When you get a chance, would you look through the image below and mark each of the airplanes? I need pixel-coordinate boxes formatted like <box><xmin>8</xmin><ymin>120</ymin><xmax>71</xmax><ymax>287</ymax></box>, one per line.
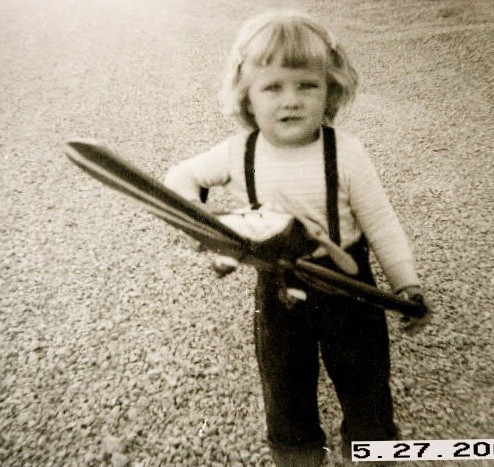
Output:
<box><xmin>61</xmin><ymin>137</ymin><xmax>429</xmax><ymax>321</ymax></box>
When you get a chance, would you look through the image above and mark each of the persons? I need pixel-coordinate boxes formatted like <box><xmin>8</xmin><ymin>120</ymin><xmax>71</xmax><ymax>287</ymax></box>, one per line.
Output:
<box><xmin>162</xmin><ymin>11</ymin><xmax>432</xmax><ymax>467</ymax></box>
